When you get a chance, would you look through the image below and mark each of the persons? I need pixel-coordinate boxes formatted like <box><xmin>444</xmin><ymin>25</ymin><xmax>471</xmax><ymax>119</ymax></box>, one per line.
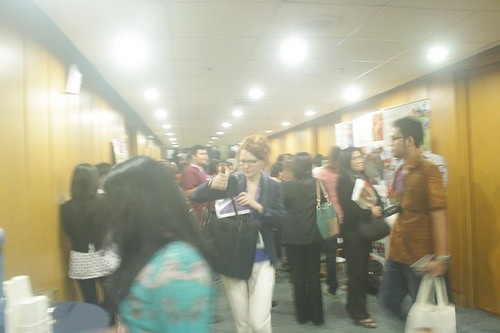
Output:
<box><xmin>60</xmin><ymin>133</ymin><xmax>384</xmax><ymax>333</ymax></box>
<box><xmin>379</xmin><ymin>116</ymin><xmax>457</xmax><ymax>333</ymax></box>
<box><xmin>103</xmin><ymin>155</ymin><xmax>219</xmax><ymax>333</ymax></box>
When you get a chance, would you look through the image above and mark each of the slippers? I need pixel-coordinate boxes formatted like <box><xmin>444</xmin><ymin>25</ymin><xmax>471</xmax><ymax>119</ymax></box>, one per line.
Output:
<box><xmin>353</xmin><ymin>315</ymin><xmax>377</xmax><ymax>329</ymax></box>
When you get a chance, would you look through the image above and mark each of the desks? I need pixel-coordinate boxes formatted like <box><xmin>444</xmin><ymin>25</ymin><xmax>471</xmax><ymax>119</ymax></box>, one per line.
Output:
<box><xmin>0</xmin><ymin>300</ymin><xmax>109</xmax><ymax>333</ymax></box>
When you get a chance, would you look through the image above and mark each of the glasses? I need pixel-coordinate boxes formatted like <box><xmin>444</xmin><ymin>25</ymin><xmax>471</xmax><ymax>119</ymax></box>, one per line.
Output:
<box><xmin>392</xmin><ymin>136</ymin><xmax>405</xmax><ymax>142</ymax></box>
<box><xmin>237</xmin><ymin>158</ymin><xmax>260</xmax><ymax>167</ymax></box>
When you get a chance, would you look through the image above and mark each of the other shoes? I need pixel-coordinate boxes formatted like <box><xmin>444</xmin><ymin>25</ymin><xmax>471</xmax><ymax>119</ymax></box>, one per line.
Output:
<box><xmin>326</xmin><ymin>286</ymin><xmax>344</xmax><ymax>296</ymax></box>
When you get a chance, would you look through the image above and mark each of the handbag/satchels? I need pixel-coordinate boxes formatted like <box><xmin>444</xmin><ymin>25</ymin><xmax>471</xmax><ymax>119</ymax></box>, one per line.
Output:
<box><xmin>315</xmin><ymin>179</ymin><xmax>340</xmax><ymax>241</ymax></box>
<box><xmin>357</xmin><ymin>217</ymin><xmax>391</xmax><ymax>241</ymax></box>
<box><xmin>404</xmin><ymin>276</ymin><xmax>457</xmax><ymax>332</ymax></box>
<box><xmin>199</xmin><ymin>191</ymin><xmax>259</xmax><ymax>280</ymax></box>
